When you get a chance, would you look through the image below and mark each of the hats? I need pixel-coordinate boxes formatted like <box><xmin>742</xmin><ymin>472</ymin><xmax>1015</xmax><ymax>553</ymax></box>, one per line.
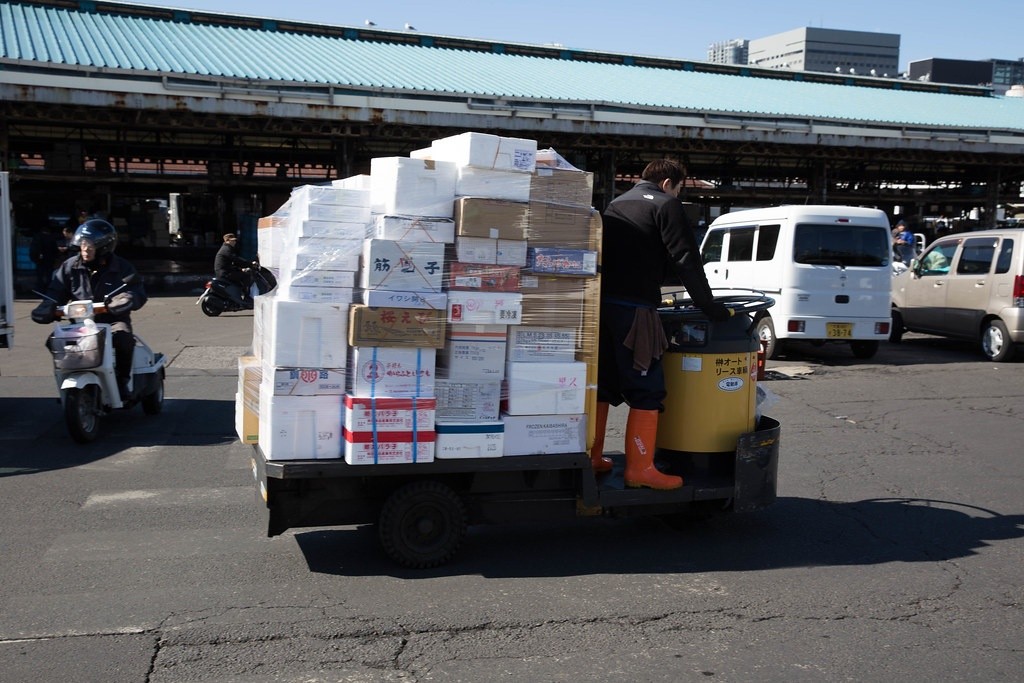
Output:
<box><xmin>223</xmin><ymin>233</ymin><xmax>237</xmax><ymax>241</ymax></box>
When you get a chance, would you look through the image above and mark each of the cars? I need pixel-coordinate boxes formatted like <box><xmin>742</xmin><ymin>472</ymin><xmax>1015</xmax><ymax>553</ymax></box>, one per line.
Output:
<box><xmin>1005</xmin><ymin>218</ymin><xmax>1024</xmax><ymax>228</ymax></box>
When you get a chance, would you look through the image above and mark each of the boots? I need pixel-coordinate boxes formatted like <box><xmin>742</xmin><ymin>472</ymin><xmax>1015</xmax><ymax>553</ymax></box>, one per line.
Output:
<box><xmin>624</xmin><ymin>408</ymin><xmax>683</xmax><ymax>490</ymax></box>
<box><xmin>112</xmin><ymin>367</ymin><xmax>133</xmax><ymax>404</ymax></box>
<box><xmin>592</xmin><ymin>402</ymin><xmax>614</xmax><ymax>471</ymax></box>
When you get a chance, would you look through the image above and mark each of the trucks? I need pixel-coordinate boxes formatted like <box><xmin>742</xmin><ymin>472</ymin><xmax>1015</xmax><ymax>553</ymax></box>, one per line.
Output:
<box><xmin>250</xmin><ymin>286</ymin><xmax>782</xmax><ymax>569</ymax></box>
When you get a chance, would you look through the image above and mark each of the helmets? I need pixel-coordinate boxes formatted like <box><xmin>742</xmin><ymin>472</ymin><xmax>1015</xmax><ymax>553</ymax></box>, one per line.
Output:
<box><xmin>77</xmin><ymin>219</ymin><xmax>117</xmax><ymax>259</ymax></box>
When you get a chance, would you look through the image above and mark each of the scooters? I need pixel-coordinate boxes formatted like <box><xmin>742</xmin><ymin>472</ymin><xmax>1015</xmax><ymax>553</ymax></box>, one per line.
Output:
<box><xmin>196</xmin><ymin>261</ymin><xmax>273</xmax><ymax>317</ymax></box>
<box><xmin>18</xmin><ymin>274</ymin><xmax>167</xmax><ymax>442</ymax></box>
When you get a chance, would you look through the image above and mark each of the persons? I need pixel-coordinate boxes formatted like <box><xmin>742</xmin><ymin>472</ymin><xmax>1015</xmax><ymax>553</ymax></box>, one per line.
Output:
<box><xmin>31</xmin><ymin>230</ymin><xmax>52</xmax><ymax>298</ymax></box>
<box><xmin>33</xmin><ymin>219</ymin><xmax>146</xmax><ymax>402</ymax></box>
<box><xmin>214</xmin><ymin>234</ymin><xmax>258</xmax><ymax>305</ymax></box>
<box><xmin>589</xmin><ymin>158</ymin><xmax>730</xmax><ymax>491</ymax></box>
<box><xmin>57</xmin><ymin>227</ymin><xmax>80</xmax><ymax>256</ymax></box>
<box><xmin>79</xmin><ymin>212</ymin><xmax>87</xmax><ymax>223</ymax></box>
<box><xmin>891</xmin><ymin>220</ymin><xmax>915</xmax><ymax>267</ymax></box>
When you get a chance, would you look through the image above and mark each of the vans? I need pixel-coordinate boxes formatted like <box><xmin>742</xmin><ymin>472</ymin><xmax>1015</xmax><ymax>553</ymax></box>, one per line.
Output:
<box><xmin>892</xmin><ymin>228</ymin><xmax>1024</xmax><ymax>362</ymax></box>
<box><xmin>681</xmin><ymin>205</ymin><xmax>893</xmax><ymax>359</ymax></box>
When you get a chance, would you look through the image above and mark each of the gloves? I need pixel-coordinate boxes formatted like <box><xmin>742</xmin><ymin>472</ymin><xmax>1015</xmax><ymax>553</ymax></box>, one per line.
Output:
<box><xmin>704</xmin><ymin>303</ymin><xmax>731</xmax><ymax>323</ymax></box>
